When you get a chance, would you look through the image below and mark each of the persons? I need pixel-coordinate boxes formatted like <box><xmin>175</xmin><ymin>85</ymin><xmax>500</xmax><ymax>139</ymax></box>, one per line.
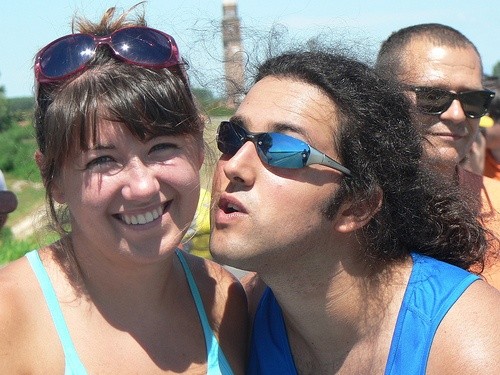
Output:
<box><xmin>374</xmin><ymin>24</ymin><xmax>500</xmax><ymax>291</ymax></box>
<box><xmin>184</xmin><ymin>18</ymin><xmax>500</xmax><ymax>375</ymax></box>
<box><xmin>0</xmin><ymin>0</ymin><xmax>249</xmax><ymax>375</ymax></box>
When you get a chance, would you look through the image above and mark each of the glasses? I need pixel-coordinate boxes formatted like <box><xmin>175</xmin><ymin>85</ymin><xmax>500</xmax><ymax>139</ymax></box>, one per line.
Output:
<box><xmin>216</xmin><ymin>121</ymin><xmax>354</xmax><ymax>177</ymax></box>
<box><xmin>485</xmin><ymin>100</ymin><xmax>500</xmax><ymax>121</ymax></box>
<box><xmin>396</xmin><ymin>83</ymin><xmax>495</xmax><ymax>119</ymax></box>
<box><xmin>34</xmin><ymin>27</ymin><xmax>193</xmax><ymax>103</ymax></box>
